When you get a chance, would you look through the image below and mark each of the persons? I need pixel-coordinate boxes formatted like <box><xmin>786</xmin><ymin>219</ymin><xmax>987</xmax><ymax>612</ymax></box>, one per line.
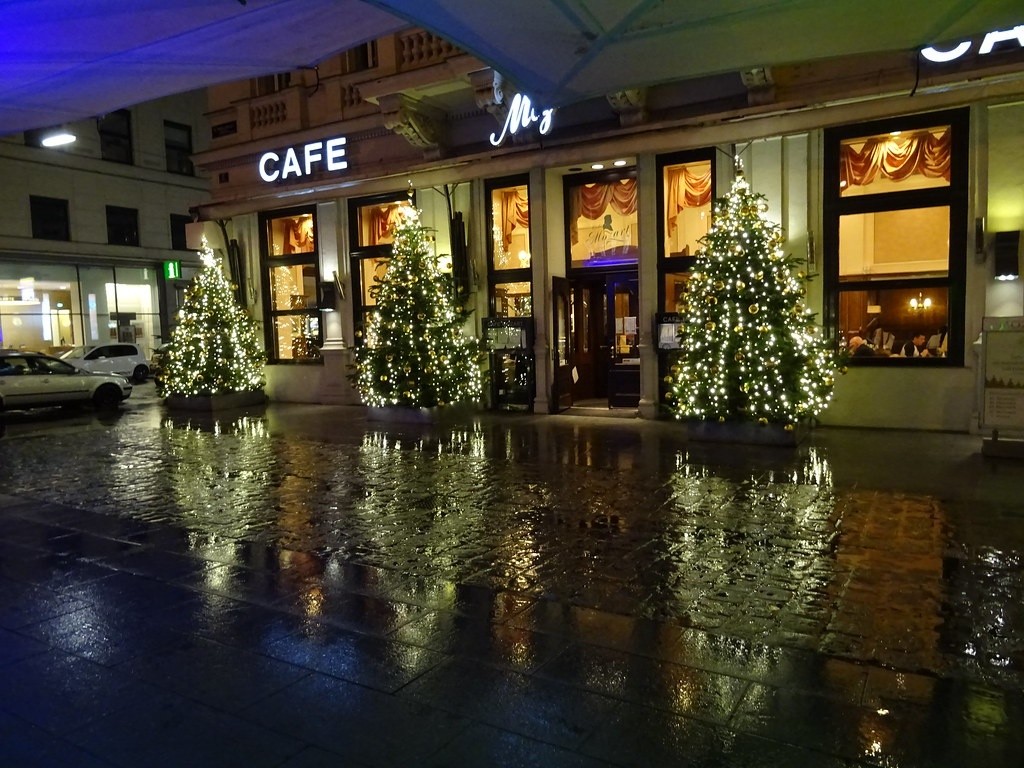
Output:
<box><xmin>849</xmin><ymin>336</ymin><xmax>875</xmax><ymax>356</ymax></box>
<box><xmin>900</xmin><ymin>332</ymin><xmax>928</xmax><ymax>358</ymax></box>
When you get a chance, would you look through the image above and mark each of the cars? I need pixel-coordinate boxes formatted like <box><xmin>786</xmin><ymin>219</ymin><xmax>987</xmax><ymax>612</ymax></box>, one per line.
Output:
<box><xmin>22</xmin><ymin>342</ymin><xmax>150</xmax><ymax>385</ymax></box>
<box><xmin>149</xmin><ymin>343</ymin><xmax>170</xmax><ymax>370</ymax></box>
<box><xmin>0</xmin><ymin>350</ymin><xmax>133</xmax><ymax>414</ymax></box>
